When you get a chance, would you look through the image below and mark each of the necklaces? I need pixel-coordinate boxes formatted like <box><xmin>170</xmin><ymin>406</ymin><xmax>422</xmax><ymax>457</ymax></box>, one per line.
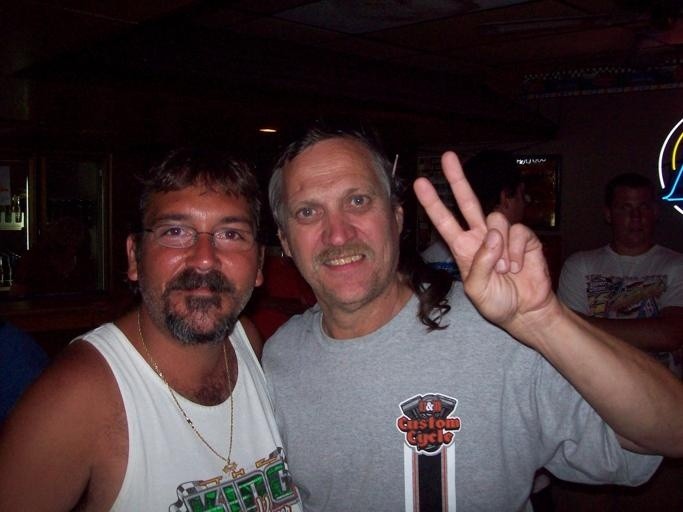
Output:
<box><xmin>137</xmin><ymin>306</ymin><xmax>237</xmax><ymax>474</ymax></box>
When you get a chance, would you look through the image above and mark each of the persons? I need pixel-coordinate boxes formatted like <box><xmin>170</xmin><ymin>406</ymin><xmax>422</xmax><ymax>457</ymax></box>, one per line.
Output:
<box><xmin>420</xmin><ymin>149</ymin><xmax>530</xmax><ymax>287</ymax></box>
<box><xmin>1</xmin><ymin>142</ymin><xmax>302</xmax><ymax>512</ymax></box>
<box><xmin>258</xmin><ymin>120</ymin><xmax>683</xmax><ymax>512</ymax></box>
<box><xmin>556</xmin><ymin>171</ymin><xmax>683</xmax><ymax>380</ymax></box>
<box><xmin>0</xmin><ymin>313</ymin><xmax>49</xmax><ymax>418</ymax></box>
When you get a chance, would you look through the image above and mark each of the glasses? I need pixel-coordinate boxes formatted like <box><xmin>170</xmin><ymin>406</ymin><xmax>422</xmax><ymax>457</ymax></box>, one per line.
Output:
<box><xmin>144</xmin><ymin>223</ymin><xmax>255</xmax><ymax>250</ymax></box>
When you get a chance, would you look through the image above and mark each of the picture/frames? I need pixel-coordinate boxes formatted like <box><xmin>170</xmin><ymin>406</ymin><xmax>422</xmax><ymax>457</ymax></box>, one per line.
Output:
<box><xmin>509</xmin><ymin>153</ymin><xmax>562</xmax><ymax>233</ymax></box>
